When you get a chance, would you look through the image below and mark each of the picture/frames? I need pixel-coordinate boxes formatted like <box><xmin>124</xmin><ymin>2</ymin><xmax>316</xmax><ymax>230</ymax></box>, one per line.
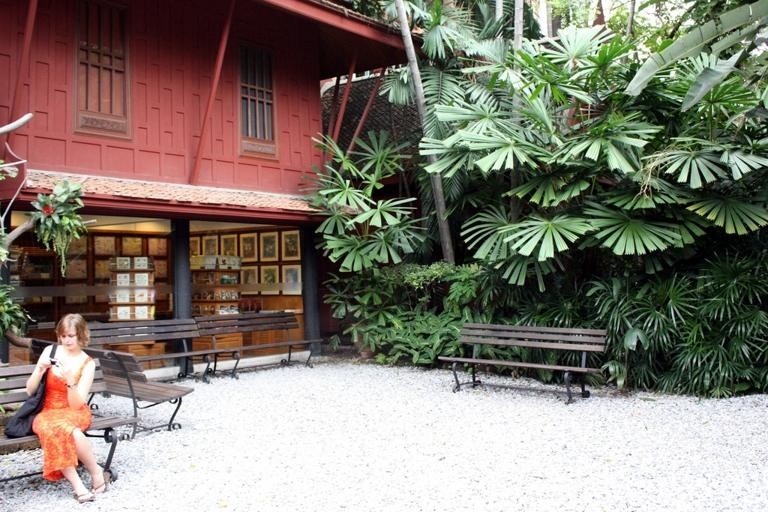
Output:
<box><xmin>57</xmin><ymin>230</ymin><xmax>304</xmax><ymax>307</ymax></box>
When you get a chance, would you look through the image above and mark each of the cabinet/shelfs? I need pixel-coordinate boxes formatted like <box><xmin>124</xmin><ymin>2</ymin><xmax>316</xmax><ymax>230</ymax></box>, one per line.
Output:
<box><xmin>184</xmin><ymin>268</ymin><xmax>249</xmax><ymax>358</ymax></box>
<box><xmin>19</xmin><ymin>246</ymin><xmax>57</xmax><ymax>341</ymax></box>
<box><xmin>102</xmin><ymin>264</ymin><xmax>170</xmax><ymax>368</ymax></box>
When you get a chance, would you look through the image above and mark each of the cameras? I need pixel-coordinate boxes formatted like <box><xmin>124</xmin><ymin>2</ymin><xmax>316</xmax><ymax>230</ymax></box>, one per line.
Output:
<box><xmin>49</xmin><ymin>359</ymin><xmax>57</xmax><ymax>365</ymax></box>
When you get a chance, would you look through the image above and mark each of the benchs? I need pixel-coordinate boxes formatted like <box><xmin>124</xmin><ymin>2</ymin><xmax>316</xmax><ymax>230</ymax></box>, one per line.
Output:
<box><xmin>19</xmin><ymin>333</ymin><xmax>195</xmax><ymax>441</ymax></box>
<box><xmin>84</xmin><ymin>314</ymin><xmax>218</xmax><ymax>383</ymax></box>
<box><xmin>435</xmin><ymin>318</ymin><xmax>610</xmax><ymax>406</ymax></box>
<box><xmin>0</xmin><ymin>356</ymin><xmax>143</xmax><ymax>483</ymax></box>
<box><xmin>192</xmin><ymin>307</ymin><xmax>326</xmax><ymax>379</ymax></box>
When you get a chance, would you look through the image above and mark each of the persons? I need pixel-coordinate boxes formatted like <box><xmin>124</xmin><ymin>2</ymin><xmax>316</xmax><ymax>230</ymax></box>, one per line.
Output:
<box><xmin>26</xmin><ymin>313</ymin><xmax>111</xmax><ymax>502</ymax></box>
<box><xmin>188</xmin><ymin>230</ymin><xmax>299</xmax><ymax>294</ymax></box>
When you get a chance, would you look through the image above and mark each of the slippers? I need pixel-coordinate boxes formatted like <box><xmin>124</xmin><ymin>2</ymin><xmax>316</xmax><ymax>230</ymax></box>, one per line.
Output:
<box><xmin>73</xmin><ymin>489</ymin><xmax>96</xmax><ymax>504</ymax></box>
<box><xmin>91</xmin><ymin>469</ymin><xmax>111</xmax><ymax>495</ymax></box>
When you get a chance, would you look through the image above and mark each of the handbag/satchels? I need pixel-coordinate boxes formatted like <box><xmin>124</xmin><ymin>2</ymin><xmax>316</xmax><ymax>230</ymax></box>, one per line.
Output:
<box><xmin>2</xmin><ymin>342</ymin><xmax>59</xmax><ymax>439</ymax></box>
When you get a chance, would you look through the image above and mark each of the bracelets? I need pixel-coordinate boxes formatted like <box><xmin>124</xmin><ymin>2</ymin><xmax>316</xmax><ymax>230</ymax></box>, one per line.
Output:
<box><xmin>64</xmin><ymin>382</ymin><xmax>79</xmax><ymax>389</ymax></box>
